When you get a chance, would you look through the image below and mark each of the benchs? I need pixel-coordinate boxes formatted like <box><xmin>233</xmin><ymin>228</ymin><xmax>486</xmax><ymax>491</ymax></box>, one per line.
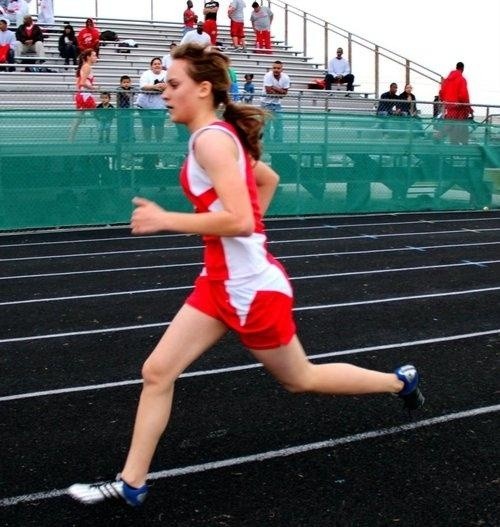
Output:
<box><xmin>1</xmin><ymin>14</ymin><xmax>426</xmax><ymax>146</ymax></box>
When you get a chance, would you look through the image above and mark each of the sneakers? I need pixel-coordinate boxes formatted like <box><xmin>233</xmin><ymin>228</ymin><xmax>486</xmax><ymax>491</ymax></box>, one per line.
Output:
<box><xmin>68</xmin><ymin>472</ymin><xmax>149</xmax><ymax>508</ymax></box>
<box><xmin>392</xmin><ymin>364</ymin><xmax>426</xmax><ymax>410</ymax></box>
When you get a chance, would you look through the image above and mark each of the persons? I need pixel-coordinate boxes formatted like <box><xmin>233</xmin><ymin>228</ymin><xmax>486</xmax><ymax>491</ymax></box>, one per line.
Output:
<box><xmin>374</xmin><ymin>61</ymin><xmax>475</xmax><ymax>148</ymax></box>
<box><xmin>76</xmin><ymin>18</ymin><xmax>102</xmax><ymax>62</ymax></box>
<box><xmin>67</xmin><ymin>48</ymin><xmax>99</xmax><ymax>143</ymax></box>
<box><xmin>115</xmin><ymin>0</ymin><xmax>291</xmax><ymax>143</ymax></box>
<box><xmin>323</xmin><ymin>47</ymin><xmax>354</xmax><ymax>96</ymax></box>
<box><xmin>64</xmin><ymin>41</ymin><xmax>434</xmax><ymax>506</ymax></box>
<box><xmin>93</xmin><ymin>92</ymin><xmax>116</xmax><ymax>146</ymax></box>
<box><xmin>0</xmin><ymin>0</ymin><xmax>55</xmax><ymax>72</ymax></box>
<box><xmin>58</xmin><ymin>25</ymin><xmax>79</xmax><ymax>71</ymax></box>
